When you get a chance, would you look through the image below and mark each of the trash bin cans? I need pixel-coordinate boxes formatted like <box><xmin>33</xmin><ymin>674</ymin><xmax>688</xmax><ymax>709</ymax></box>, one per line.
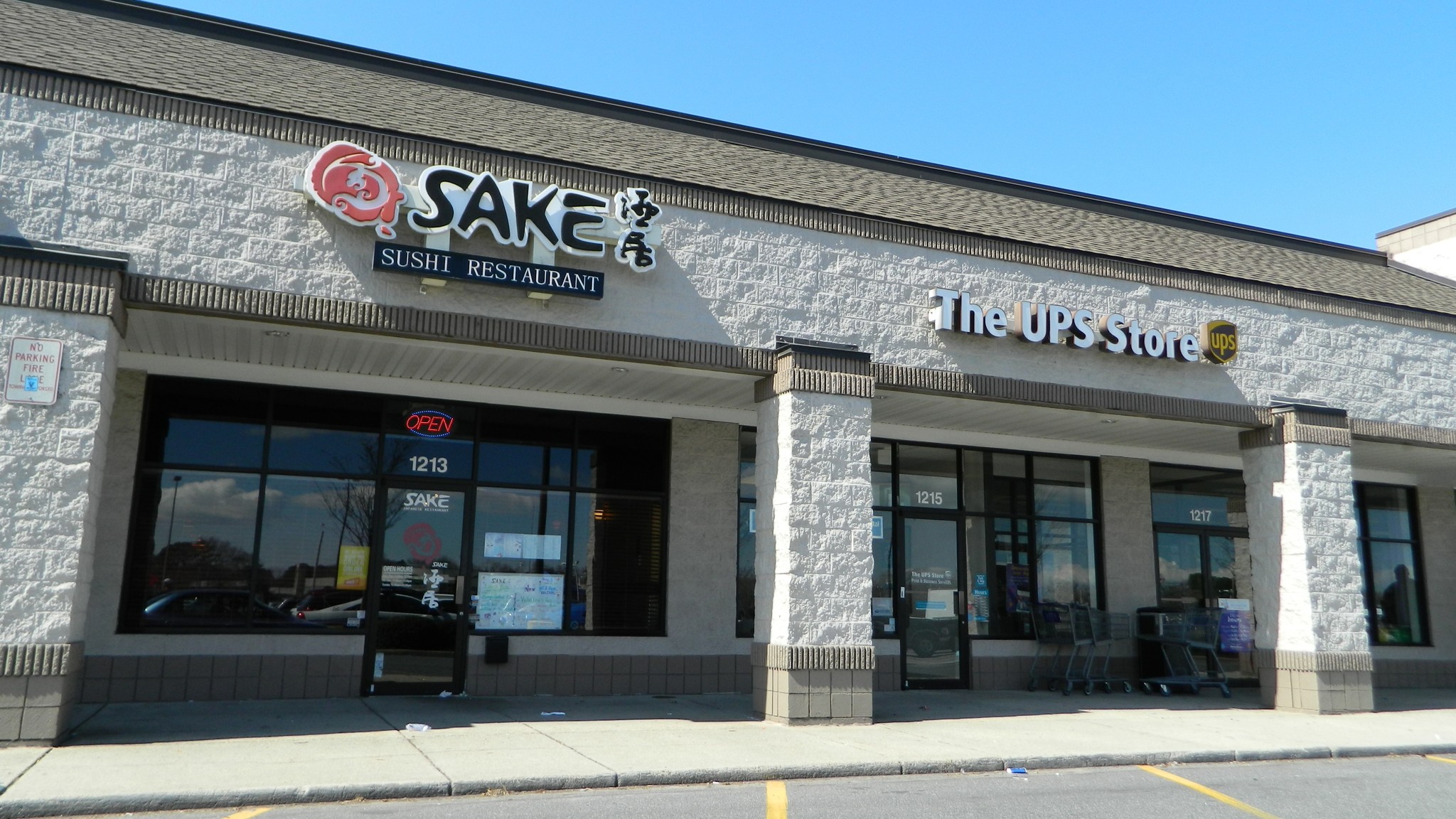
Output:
<box><xmin>1137</xmin><ymin>607</ymin><xmax>1188</xmax><ymax>693</ymax></box>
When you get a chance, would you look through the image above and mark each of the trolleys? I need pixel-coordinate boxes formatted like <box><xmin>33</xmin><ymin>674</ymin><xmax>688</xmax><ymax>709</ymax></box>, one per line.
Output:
<box><xmin>1135</xmin><ymin>608</ymin><xmax>1232</xmax><ymax>698</ymax></box>
<box><xmin>1063</xmin><ymin>602</ymin><xmax>1134</xmax><ymax>696</ymax></box>
<box><xmin>1026</xmin><ymin>601</ymin><xmax>1080</xmax><ymax>691</ymax></box>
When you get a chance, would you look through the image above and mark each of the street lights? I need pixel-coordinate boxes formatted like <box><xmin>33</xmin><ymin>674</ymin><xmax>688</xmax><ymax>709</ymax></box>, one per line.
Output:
<box><xmin>312</xmin><ymin>523</ymin><xmax>325</xmax><ymax>588</ymax></box>
<box><xmin>159</xmin><ymin>476</ymin><xmax>182</xmax><ymax>592</ymax></box>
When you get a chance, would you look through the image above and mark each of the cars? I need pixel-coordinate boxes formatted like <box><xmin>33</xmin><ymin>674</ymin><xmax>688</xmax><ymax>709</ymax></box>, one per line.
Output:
<box><xmin>471</xmin><ymin>594</ymin><xmax>586</xmax><ymax>631</ymax></box>
<box><xmin>143</xmin><ymin>590</ymin><xmax>292</xmax><ymax>628</ymax></box>
<box><xmin>269</xmin><ymin>588</ymin><xmax>509</xmax><ymax>655</ymax></box>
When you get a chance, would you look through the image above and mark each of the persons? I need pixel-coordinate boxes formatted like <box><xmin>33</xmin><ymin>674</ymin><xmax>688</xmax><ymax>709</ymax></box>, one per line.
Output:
<box><xmin>1381</xmin><ymin>564</ymin><xmax>1418</xmax><ymax>643</ymax></box>
